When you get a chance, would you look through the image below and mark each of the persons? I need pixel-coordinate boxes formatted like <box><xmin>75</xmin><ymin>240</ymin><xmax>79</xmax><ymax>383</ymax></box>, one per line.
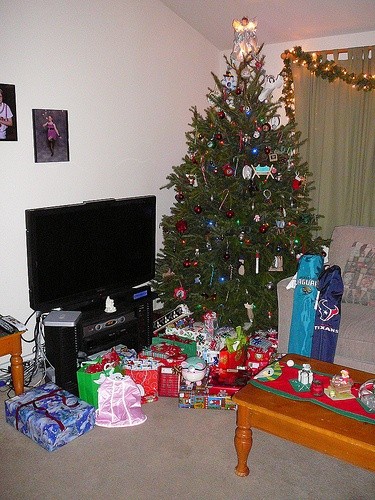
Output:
<box><xmin>0</xmin><ymin>89</ymin><xmax>13</xmax><ymax>140</ymax></box>
<box><xmin>43</xmin><ymin>115</ymin><xmax>59</xmax><ymax>156</ymax></box>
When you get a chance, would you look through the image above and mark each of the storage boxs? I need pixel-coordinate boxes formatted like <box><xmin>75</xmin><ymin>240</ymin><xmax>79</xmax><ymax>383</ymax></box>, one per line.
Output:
<box><xmin>5</xmin><ymin>313</ymin><xmax>275</xmax><ymax>453</ymax></box>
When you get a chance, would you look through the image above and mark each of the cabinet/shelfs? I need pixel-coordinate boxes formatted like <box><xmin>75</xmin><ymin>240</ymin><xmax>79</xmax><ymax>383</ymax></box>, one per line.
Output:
<box><xmin>77</xmin><ymin>293</ymin><xmax>160</xmax><ymax>354</ymax></box>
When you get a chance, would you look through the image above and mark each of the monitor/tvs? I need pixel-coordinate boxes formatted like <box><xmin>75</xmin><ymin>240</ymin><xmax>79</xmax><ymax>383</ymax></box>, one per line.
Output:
<box><xmin>26</xmin><ymin>195</ymin><xmax>156</xmax><ymax>311</ymax></box>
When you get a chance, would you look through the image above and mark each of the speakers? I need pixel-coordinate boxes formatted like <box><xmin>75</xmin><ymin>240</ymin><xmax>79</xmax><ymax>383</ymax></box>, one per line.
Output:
<box><xmin>43</xmin><ymin>320</ymin><xmax>83</xmax><ymax>387</ymax></box>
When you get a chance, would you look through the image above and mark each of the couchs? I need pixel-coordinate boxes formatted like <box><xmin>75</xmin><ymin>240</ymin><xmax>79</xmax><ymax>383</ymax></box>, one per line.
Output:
<box><xmin>277</xmin><ymin>226</ymin><xmax>375</xmax><ymax>373</ymax></box>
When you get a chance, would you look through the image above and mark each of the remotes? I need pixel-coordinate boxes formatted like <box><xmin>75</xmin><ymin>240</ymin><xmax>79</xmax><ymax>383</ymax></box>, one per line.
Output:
<box><xmin>0</xmin><ymin>319</ymin><xmax>14</xmax><ymax>333</ymax></box>
<box><xmin>1</xmin><ymin>316</ymin><xmax>26</xmax><ymax>331</ymax></box>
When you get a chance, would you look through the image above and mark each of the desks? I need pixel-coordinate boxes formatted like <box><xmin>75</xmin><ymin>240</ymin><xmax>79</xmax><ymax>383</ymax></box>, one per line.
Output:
<box><xmin>231</xmin><ymin>353</ymin><xmax>375</xmax><ymax>477</ymax></box>
<box><xmin>0</xmin><ymin>313</ymin><xmax>26</xmax><ymax>398</ymax></box>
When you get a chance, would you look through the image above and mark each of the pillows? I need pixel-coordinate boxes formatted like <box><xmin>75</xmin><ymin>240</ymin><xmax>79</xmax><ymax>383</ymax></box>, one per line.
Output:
<box><xmin>340</xmin><ymin>241</ymin><xmax>375</xmax><ymax>307</ymax></box>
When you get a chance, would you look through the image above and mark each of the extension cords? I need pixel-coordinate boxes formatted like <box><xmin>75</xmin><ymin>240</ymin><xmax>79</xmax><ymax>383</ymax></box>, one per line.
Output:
<box><xmin>44</xmin><ymin>367</ymin><xmax>56</xmax><ymax>383</ymax></box>
